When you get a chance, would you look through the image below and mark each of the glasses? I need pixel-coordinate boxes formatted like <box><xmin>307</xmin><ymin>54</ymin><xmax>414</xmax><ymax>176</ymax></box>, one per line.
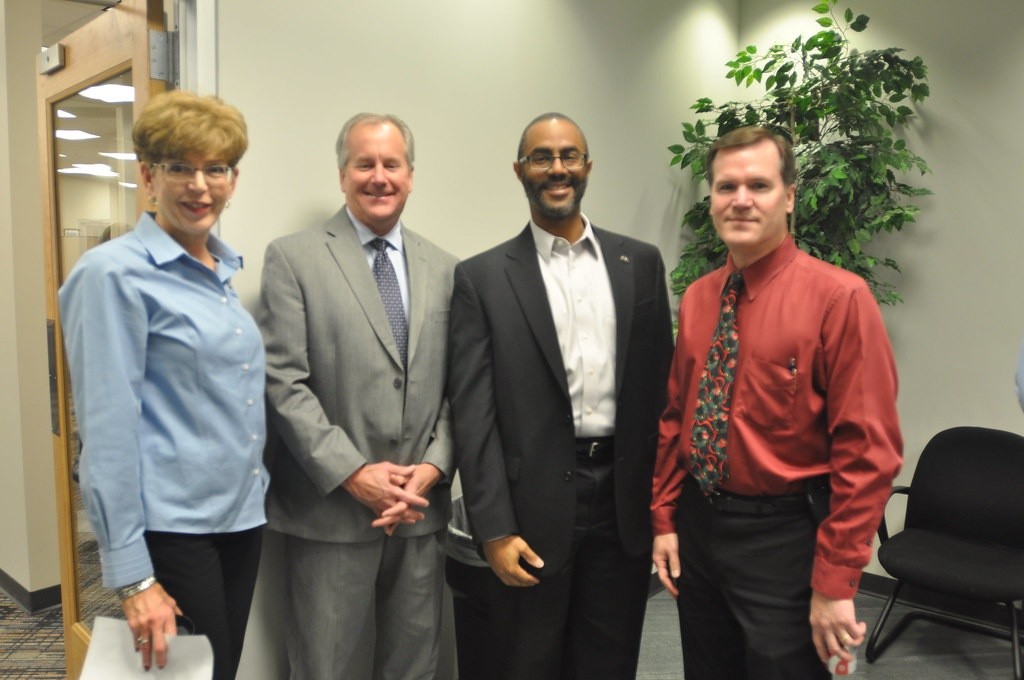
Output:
<box><xmin>151</xmin><ymin>159</ymin><xmax>237</xmax><ymax>186</ymax></box>
<box><xmin>517</xmin><ymin>153</ymin><xmax>591</xmax><ymax>173</ymax></box>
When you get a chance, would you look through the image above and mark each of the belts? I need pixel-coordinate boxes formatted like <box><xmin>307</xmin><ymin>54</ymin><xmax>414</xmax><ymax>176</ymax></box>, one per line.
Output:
<box><xmin>687</xmin><ymin>477</ymin><xmax>831</xmax><ymax>515</ymax></box>
<box><xmin>577</xmin><ymin>439</ymin><xmax>615</xmax><ymax>458</ymax></box>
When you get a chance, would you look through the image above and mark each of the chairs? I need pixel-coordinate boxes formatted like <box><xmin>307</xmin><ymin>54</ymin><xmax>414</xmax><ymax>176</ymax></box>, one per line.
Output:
<box><xmin>865</xmin><ymin>426</ymin><xmax>1024</xmax><ymax>680</ymax></box>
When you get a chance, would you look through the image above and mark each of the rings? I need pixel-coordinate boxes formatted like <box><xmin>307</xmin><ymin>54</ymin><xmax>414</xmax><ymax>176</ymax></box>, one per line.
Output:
<box><xmin>840</xmin><ymin>635</ymin><xmax>850</xmax><ymax>642</ymax></box>
<box><xmin>137</xmin><ymin>637</ymin><xmax>150</xmax><ymax>645</ymax></box>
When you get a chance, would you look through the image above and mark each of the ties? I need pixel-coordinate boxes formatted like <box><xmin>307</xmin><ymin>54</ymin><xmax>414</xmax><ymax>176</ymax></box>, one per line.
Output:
<box><xmin>689</xmin><ymin>275</ymin><xmax>746</xmax><ymax>508</ymax></box>
<box><xmin>368</xmin><ymin>238</ymin><xmax>409</xmax><ymax>411</ymax></box>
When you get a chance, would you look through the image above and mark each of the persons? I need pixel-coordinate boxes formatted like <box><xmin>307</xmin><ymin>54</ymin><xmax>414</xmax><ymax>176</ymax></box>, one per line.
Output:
<box><xmin>259</xmin><ymin>114</ymin><xmax>459</xmax><ymax>680</ymax></box>
<box><xmin>653</xmin><ymin>128</ymin><xmax>905</xmax><ymax>679</ymax></box>
<box><xmin>59</xmin><ymin>95</ymin><xmax>270</xmax><ymax>680</ymax></box>
<box><xmin>449</xmin><ymin>114</ymin><xmax>674</xmax><ymax>679</ymax></box>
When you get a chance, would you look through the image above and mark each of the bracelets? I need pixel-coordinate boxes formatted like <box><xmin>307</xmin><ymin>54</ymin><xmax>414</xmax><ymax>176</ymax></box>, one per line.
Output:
<box><xmin>115</xmin><ymin>576</ymin><xmax>156</xmax><ymax>600</ymax></box>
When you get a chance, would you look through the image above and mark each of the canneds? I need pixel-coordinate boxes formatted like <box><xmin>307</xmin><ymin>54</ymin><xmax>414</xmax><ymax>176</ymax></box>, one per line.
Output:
<box><xmin>827</xmin><ymin>637</ymin><xmax>858</xmax><ymax>676</ymax></box>
